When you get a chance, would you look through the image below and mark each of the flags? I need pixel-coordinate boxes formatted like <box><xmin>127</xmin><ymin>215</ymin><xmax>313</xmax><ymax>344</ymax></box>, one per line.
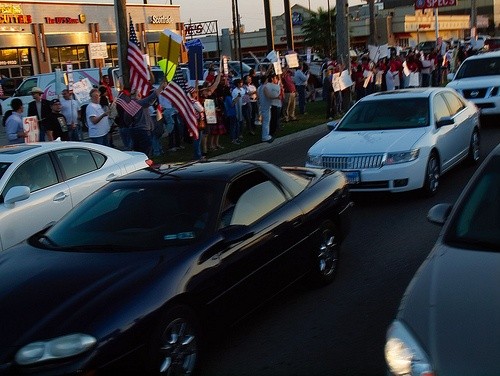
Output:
<box><xmin>160</xmin><ymin>81</ymin><xmax>200</xmax><ymax>141</ymax></box>
<box><xmin>174</xmin><ymin>62</ymin><xmax>196</xmax><ymax>94</ymax></box>
<box><xmin>127</xmin><ymin>13</ymin><xmax>150</xmax><ymax>98</ymax></box>
<box><xmin>114</xmin><ymin>92</ymin><xmax>142</xmax><ymax>117</ymax></box>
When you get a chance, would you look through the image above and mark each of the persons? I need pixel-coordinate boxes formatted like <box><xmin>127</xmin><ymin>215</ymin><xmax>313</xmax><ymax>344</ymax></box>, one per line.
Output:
<box><xmin>47</xmin><ymin>99</ymin><xmax>75</xmax><ymax>142</ymax></box>
<box><xmin>5</xmin><ymin>99</ymin><xmax>29</xmax><ymax>145</ymax></box>
<box><xmin>322</xmin><ymin>40</ymin><xmax>490</xmax><ymax>120</ymax></box>
<box><xmin>96</xmin><ymin>69</ymin><xmax>168</xmax><ymax>169</ymax></box>
<box><xmin>86</xmin><ymin>89</ymin><xmax>113</xmax><ymax>147</ymax></box>
<box><xmin>185</xmin><ymin>64</ymin><xmax>310</xmax><ymax>162</ymax></box>
<box><xmin>28</xmin><ymin>87</ymin><xmax>53</xmax><ymax>142</ymax></box>
<box><xmin>58</xmin><ymin>89</ymin><xmax>78</xmax><ymax>141</ymax></box>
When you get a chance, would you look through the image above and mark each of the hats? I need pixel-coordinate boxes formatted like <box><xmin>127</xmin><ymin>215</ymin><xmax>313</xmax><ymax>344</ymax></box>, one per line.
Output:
<box><xmin>50</xmin><ymin>99</ymin><xmax>61</xmax><ymax>105</ymax></box>
<box><xmin>28</xmin><ymin>87</ymin><xmax>44</xmax><ymax>94</ymax></box>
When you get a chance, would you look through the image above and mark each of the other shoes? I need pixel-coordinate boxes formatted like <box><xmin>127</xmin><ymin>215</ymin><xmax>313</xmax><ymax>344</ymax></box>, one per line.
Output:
<box><xmin>216</xmin><ymin>145</ymin><xmax>224</xmax><ymax>149</ymax></box>
<box><xmin>265</xmin><ymin>138</ymin><xmax>272</xmax><ymax>143</ymax></box>
<box><xmin>202</xmin><ymin>151</ymin><xmax>212</xmax><ymax>156</ymax></box>
<box><xmin>290</xmin><ymin>116</ymin><xmax>298</xmax><ymax>120</ymax></box>
<box><xmin>285</xmin><ymin>117</ymin><xmax>289</xmax><ymax>123</ymax></box>
<box><xmin>210</xmin><ymin>147</ymin><xmax>219</xmax><ymax>151</ymax></box>
<box><xmin>233</xmin><ymin>139</ymin><xmax>241</xmax><ymax>145</ymax></box>
